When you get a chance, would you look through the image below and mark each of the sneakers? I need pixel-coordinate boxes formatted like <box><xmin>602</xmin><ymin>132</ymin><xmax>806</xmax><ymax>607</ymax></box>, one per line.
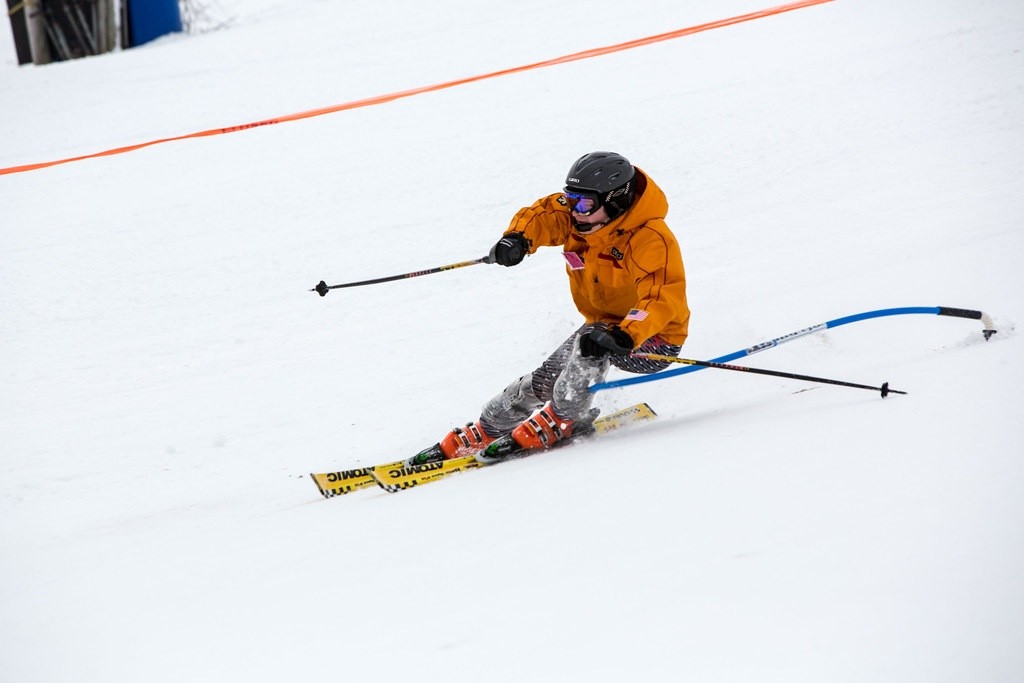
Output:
<box><xmin>441</xmin><ymin>422</ymin><xmax>498</xmax><ymax>460</ymax></box>
<box><xmin>512</xmin><ymin>406</ymin><xmax>573</xmax><ymax>451</ymax></box>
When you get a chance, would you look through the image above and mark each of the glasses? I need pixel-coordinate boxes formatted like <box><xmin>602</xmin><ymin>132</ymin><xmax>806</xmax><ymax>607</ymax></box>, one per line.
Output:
<box><xmin>563</xmin><ymin>187</ymin><xmax>601</xmax><ymax>216</ymax></box>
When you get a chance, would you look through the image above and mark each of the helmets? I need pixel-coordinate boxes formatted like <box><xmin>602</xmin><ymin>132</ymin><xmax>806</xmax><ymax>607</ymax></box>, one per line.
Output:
<box><xmin>566</xmin><ymin>151</ymin><xmax>635</xmax><ymax>219</ymax></box>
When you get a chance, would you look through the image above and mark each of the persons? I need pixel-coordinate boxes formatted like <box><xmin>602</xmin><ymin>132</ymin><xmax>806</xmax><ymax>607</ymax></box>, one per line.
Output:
<box><xmin>442</xmin><ymin>152</ymin><xmax>691</xmax><ymax>461</ymax></box>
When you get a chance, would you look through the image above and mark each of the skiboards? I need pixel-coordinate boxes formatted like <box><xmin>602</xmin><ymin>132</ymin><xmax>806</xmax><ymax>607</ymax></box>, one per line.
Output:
<box><xmin>310</xmin><ymin>403</ymin><xmax>658</xmax><ymax>499</ymax></box>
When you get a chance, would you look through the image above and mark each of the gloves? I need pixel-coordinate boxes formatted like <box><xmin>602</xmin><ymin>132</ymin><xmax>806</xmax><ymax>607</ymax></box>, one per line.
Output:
<box><xmin>579</xmin><ymin>327</ymin><xmax>634</xmax><ymax>359</ymax></box>
<box><xmin>495</xmin><ymin>233</ymin><xmax>528</xmax><ymax>267</ymax></box>
